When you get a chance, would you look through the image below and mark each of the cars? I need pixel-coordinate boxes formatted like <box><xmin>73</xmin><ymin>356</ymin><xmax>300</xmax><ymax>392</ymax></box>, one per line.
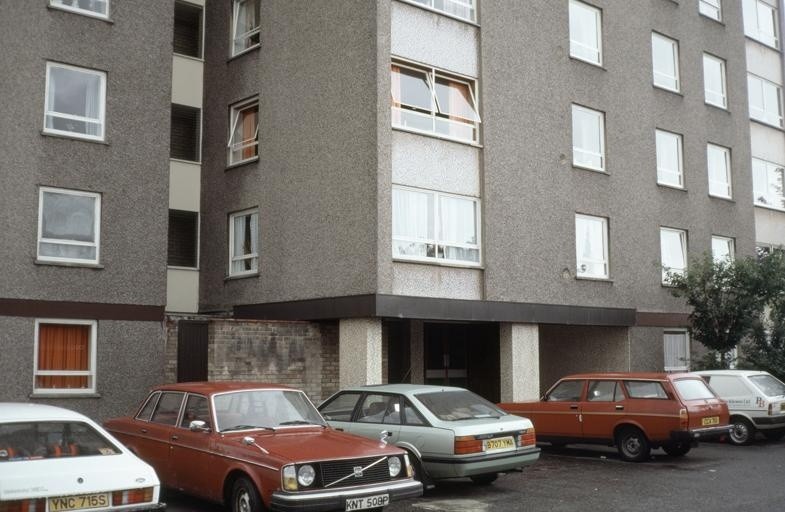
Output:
<box><xmin>0</xmin><ymin>402</ymin><xmax>167</xmax><ymax>512</ymax></box>
<box><xmin>314</xmin><ymin>382</ymin><xmax>542</xmax><ymax>498</ymax></box>
<box><xmin>495</xmin><ymin>371</ymin><xmax>735</xmax><ymax>463</ymax></box>
<box><xmin>702</xmin><ymin>366</ymin><xmax>784</xmax><ymax>443</ymax></box>
<box><xmin>100</xmin><ymin>381</ymin><xmax>425</xmax><ymax>512</ymax></box>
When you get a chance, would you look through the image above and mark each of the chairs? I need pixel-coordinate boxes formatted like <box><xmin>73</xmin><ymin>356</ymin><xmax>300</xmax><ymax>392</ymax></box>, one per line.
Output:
<box><xmin>366</xmin><ymin>402</ymin><xmax>384</xmax><ymax>415</ymax></box>
